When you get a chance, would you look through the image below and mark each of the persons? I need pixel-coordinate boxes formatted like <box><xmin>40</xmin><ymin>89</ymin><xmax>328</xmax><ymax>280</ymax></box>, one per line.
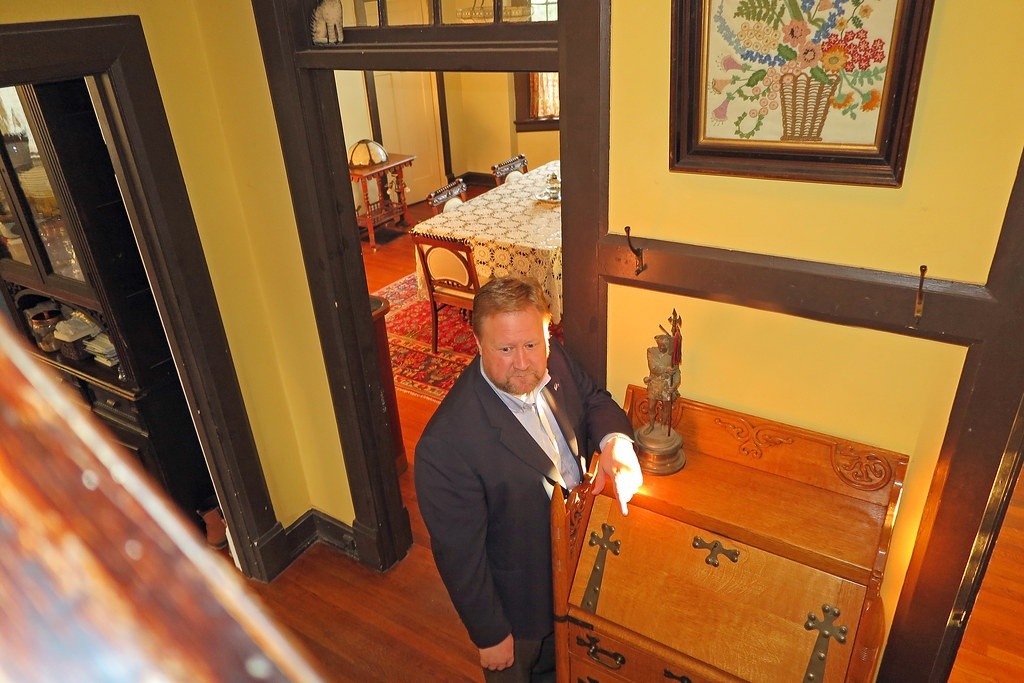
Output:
<box><xmin>416</xmin><ymin>276</ymin><xmax>644</xmax><ymax>683</ymax></box>
<box><xmin>641</xmin><ymin>335</ymin><xmax>681</xmax><ymax>436</ymax></box>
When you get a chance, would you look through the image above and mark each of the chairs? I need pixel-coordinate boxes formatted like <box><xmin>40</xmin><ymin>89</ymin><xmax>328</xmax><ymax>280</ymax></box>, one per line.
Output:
<box><xmin>491</xmin><ymin>153</ymin><xmax>529</xmax><ymax>187</ymax></box>
<box><xmin>409</xmin><ymin>230</ymin><xmax>480</xmax><ymax>353</ymax></box>
<box><xmin>426</xmin><ymin>178</ymin><xmax>467</xmax><ymax>216</ymax></box>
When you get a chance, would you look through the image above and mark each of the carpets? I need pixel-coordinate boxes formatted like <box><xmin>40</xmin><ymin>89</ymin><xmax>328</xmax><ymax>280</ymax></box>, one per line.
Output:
<box><xmin>370</xmin><ymin>272</ymin><xmax>564</xmax><ymax>405</ymax></box>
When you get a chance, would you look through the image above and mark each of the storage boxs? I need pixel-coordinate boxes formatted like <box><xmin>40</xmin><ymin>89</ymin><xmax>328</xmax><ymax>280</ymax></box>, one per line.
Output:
<box><xmin>54</xmin><ymin>334</ymin><xmax>95</xmax><ymax>360</ymax></box>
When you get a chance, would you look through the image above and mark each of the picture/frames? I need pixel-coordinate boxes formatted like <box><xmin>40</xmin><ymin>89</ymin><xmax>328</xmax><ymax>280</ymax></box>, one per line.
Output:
<box><xmin>668</xmin><ymin>0</ymin><xmax>935</xmax><ymax>190</ymax></box>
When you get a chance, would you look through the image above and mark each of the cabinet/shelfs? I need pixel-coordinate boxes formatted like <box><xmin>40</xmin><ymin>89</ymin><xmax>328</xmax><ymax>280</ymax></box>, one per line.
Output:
<box><xmin>0</xmin><ymin>78</ymin><xmax>218</xmax><ymax>513</ymax></box>
<box><xmin>551</xmin><ymin>384</ymin><xmax>911</xmax><ymax>683</ymax></box>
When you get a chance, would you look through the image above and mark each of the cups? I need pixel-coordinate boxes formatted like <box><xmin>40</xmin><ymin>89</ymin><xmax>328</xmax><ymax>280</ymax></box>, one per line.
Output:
<box><xmin>546</xmin><ymin>186</ymin><xmax>560</xmax><ymax>199</ymax></box>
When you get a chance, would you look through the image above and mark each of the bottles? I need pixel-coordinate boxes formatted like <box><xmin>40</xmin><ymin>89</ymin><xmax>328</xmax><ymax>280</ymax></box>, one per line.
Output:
<box><xmin>31</xmin><ymin>309</ymin><xmax>67</xmax><ymax>353</ymax></box>
<box><xmin>1</xmin><ymin>209</ymin><xmax>34</xmax><ymax>266</ymax></box>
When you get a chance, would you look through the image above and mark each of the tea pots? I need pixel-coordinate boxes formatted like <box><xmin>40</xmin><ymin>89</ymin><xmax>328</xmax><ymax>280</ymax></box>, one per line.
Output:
<box><xmin>546</xmin><ymin>172</ymin><xmax>562</xmax><ymax>185</ymax></box>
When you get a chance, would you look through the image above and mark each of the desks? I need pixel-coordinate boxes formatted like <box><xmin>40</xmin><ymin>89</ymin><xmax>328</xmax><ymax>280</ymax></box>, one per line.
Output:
<box><xmin>414</xmin><ymin>159</ymin><xmax>564</xmax><ymax>325</ymax></box>
<box><xmin>348</xmin><ymin>153</ymin><xmax>418</xmax><ymax>249</ymax></box>
<box><xmin>369</xmin><ymin>294</ymin><xmax>408</xmax><ymax>478</ymax></box>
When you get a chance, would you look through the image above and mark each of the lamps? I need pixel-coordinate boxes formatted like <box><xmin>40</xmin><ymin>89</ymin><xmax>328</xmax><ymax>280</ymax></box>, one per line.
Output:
<box><xmin>456</xmin><ymin>0</ymin><xmax>534</xmax><ymax>23</ymax></box>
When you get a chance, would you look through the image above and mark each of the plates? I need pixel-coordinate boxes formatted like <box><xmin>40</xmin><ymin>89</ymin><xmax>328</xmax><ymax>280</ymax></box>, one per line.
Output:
<box><xmin>17</xmin><ymin>165</ymin><xmax>55</xmax><ymax>198</ymax></box>
<box><xmin>536</xmin><ymin>190</ymin><xmax>561</xmax><ymax>204</ymax></box>
